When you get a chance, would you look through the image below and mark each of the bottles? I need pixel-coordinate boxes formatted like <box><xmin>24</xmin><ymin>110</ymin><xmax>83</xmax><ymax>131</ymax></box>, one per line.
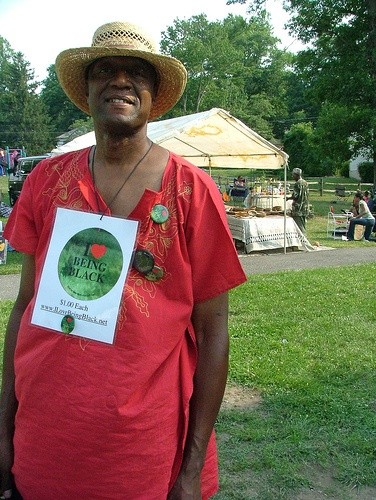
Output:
<box><xmin>330</xmin><ymin>205</ymin><xmax>334</xmax><ymax>215</ymax></box>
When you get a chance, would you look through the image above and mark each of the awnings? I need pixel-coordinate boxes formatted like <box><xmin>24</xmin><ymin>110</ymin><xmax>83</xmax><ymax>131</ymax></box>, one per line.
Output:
<box><xmin>47</xmin><ymin>108</ymin><xmax>290</xmax><ymax>254</ymax></box>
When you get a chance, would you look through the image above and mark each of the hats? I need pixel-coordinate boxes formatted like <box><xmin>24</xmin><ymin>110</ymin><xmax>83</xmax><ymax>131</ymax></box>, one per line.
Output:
<box><xmin>56</xmin><ymin>23</ymin><xmax>187</xmax><ymax>120</ymax></box>
<box><xmin>293</xmin><ymin>167</ymin><xmax>302</xmax><ymax>175</ymax></box>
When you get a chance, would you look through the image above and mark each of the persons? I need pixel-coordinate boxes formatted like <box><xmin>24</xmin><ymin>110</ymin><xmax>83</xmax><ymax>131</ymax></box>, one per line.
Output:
<box><xmin>284</xmin><ymin>168</ymin><xmax>309</xmax><ymax>240</ymax></box>
<box><xmin>344</xmin><ymin>189</ymin><xmax>376</xmax><ymax>243</ymax></box>
<box><xmin>0</xmin><ymin>22</ymin><xmax>248</xmax><ymax>500</ymax></box>
<box><xmin>0</xmin><ymin>150</ymin><xmax>19</xmax><ymax>176</ymax></box>
<box><xmin>234</xmin><ymin>175</ymin><xmax>244</xmax><ymax>188</ymax></box>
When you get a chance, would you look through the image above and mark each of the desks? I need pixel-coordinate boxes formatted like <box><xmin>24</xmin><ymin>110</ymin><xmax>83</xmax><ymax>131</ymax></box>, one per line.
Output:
<box><xmin>252</xmin><ymin>195</ymin><xmax>293</xmax><ymax>212</ymax></box>
<box><xmin>224</xmin><ymin>205</ymin><xmax>298</xmax><ymax>254</ymax></box>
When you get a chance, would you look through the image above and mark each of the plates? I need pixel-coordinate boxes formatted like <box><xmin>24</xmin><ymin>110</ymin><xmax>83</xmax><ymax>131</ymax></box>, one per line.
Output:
<box><xmin>226</xmin><ymin>206</ymin><xmax>284</xmax><ymax>218</ymax></box>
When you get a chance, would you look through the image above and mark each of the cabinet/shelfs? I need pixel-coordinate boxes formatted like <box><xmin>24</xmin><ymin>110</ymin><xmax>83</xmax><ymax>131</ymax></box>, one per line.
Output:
<box><xmin>327</xmin><ymin>212</ymin><xmax>353</xmax><ymax>241</ymax></box>
